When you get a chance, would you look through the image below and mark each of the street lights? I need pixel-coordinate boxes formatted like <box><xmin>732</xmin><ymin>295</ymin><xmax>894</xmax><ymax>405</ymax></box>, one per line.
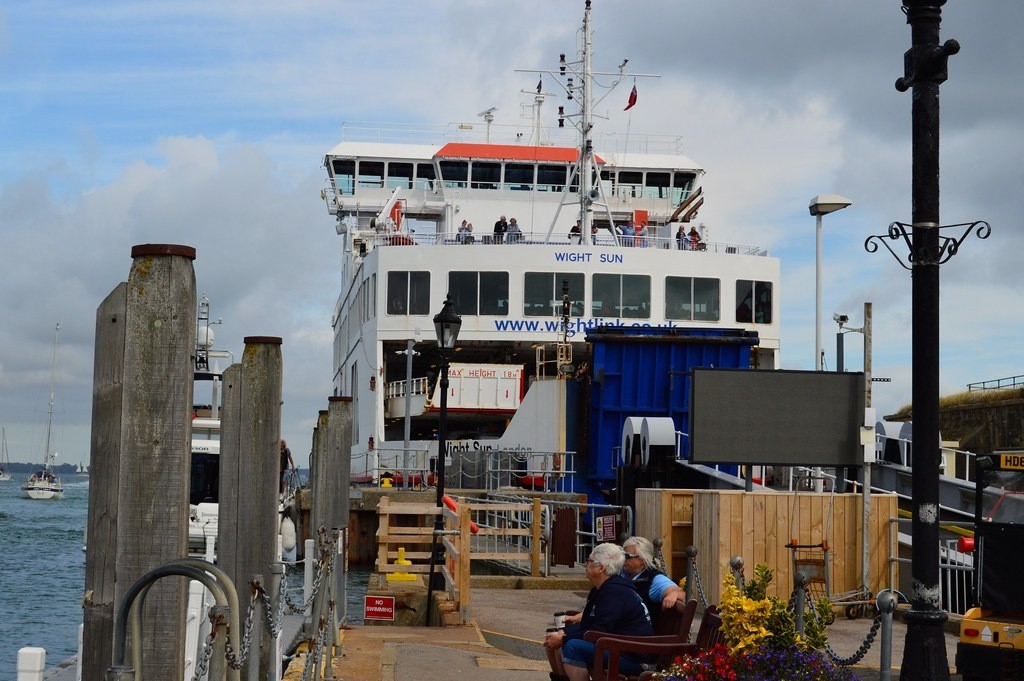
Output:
<box><xmin>423</xmin><ymin>292</ymin><xmax>462</xmax><ymax>625</ymax></box>
<box><xmin>808</xmin><ymin>195</ymin><xmax>854</xmax><ymax>371</ymax></box>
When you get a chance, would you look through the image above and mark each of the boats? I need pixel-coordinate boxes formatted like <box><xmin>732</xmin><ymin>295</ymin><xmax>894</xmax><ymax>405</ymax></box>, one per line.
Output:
<box><xmin>323</xmin><ymin>1</ymin><xmax>781</xmax><ymax>490</ymax></box>
<box><xmin>187</xmin><ymin>290</ymin><xmax>284</xmax><ymax>548</ymax></box>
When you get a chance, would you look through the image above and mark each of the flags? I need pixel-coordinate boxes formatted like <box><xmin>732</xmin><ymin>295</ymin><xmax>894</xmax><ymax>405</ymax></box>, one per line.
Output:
<box><xmin>623</xmin><ymin>85</ymin><xmax>637</xmax><ymax>112</ymax></box>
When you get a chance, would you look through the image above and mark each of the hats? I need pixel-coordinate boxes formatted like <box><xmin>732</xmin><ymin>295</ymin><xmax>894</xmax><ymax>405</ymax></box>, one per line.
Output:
<box><xmin>576</xmin><ymin>220</ymin><xmax>582</xmax><ymax>223</ymax></box>
<box><xmin>462</xmin><ymin>220</ymin><xmax>467</xmax><ymax>223</ymax></box>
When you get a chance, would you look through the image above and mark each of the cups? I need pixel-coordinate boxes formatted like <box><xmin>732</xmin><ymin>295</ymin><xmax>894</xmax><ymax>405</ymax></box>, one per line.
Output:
<box><xmin>554</xmin><ymin>611</ymin><xmax>565</xmax><ymax>629</ymax></box>
<box><xmin>546</xmin><ymin>628</ymin><xmax>559</xmax><ymax>640</ymax></box>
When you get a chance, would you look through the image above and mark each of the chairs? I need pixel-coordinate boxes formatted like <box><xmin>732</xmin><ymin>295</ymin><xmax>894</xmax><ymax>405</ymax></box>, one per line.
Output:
<box><xmin>584</xmin><ymin>599</ymin><xmax>735</xmax><ymax>681</ymax></box>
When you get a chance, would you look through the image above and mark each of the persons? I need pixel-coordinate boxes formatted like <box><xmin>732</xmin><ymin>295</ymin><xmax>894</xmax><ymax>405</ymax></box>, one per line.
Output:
<box><xmin>506</xmin><ymin>218</ymin><xmax>520</xmax><ymax>242</ymax></box>
<box><xmin>638</xmin><ymin>221</ymin><xmax>649</xmax><ymax>248</ymax></box>
<box><xmin>458</xmin><ymin>220</ymin><xmax>473</xmax><ymax>244</ymax></box>
<box><xmin>676</xmin><ymin>226</ymin><xmax>688</xmax><ymax>250</ymax></box>
<box><xmin>491</xmin><ymin>216</ymin><xmax>508</xmax><ymax>244</ymax></box>
<box><xmin>554</xmin><ymin>534</ymin><xmax>680</xmax><ymax>681</ymax></box>
<box><xmin>618</xmin><ymin>220</ymin><xmax>636</xmax><ymax>247</ymax></box>
<box><xmin>280</xmin><ymin>439</ymin><xmax>296</xmax><ymax>501</ymax></box>
<box><xmin>687</xmin><ymin>227</ymin><xmax>701</xmax><ymax>251</ymax></box>
<box><xmin>543</xmin><ymin>543</ymin><xmax>658</xmax><ymax>681</ymax></box>
<box><xmin>590</xmin><ymin>223</ymin><xmax>598</xmax><ymax>246</ymax></box>
<box><xmin>569</xmin><ymin>219</ymin><xmax>582</xmax><ymax>245</ymax></box>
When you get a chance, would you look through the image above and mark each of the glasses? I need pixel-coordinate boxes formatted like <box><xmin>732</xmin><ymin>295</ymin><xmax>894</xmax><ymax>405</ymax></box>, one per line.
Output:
<box><xmin>625</xmin><ymin>553</ymin><xmax>638</xmax><ymax>559</ymax></box>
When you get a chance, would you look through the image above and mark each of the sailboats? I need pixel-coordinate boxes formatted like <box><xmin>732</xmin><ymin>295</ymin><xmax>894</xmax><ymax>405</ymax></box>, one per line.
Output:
<box><xmin>75</xmin><ymin>458</ymin><xmax>90</xmax><ymax>478</ymax></box>
<box><xmin>0</xmin><ymin>426</ymin><xmax>12</xmax><ymax>481</ymax></box>
<box><xmin>19</xmin><ymin>318</ymin><xmax>66</xmax><ymax>500</ymax></box>
